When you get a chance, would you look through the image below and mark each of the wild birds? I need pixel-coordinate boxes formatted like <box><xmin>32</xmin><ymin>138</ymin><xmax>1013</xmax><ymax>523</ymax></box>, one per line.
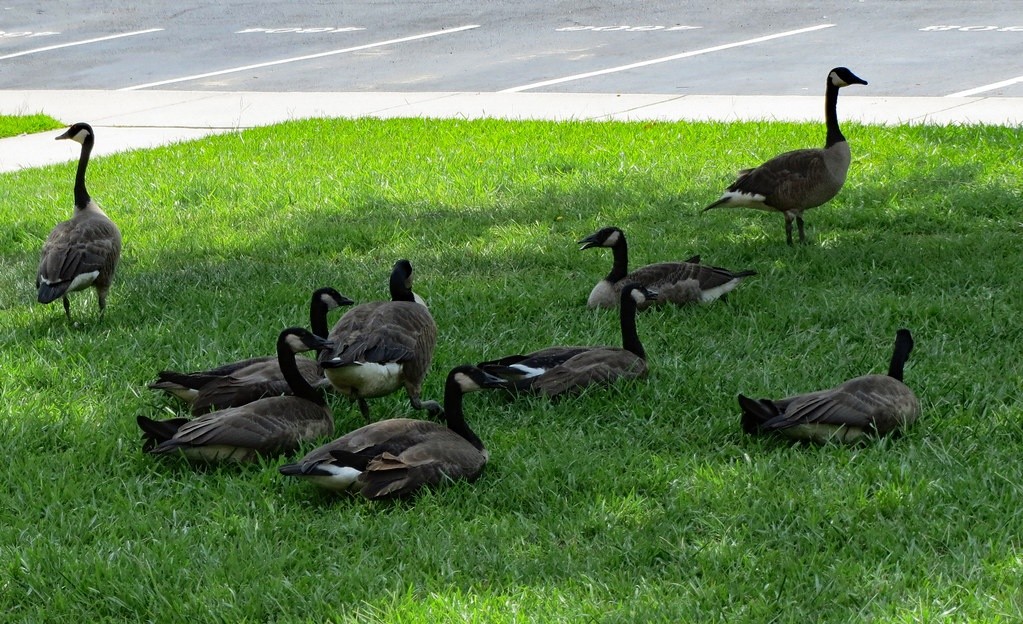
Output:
<box><xmin>316</xmin><ymin>259</ymin><xmax>444</xmax><ymax>423</ymax></box>
<box><xmin>454</xmin><ymin>282</ymin><xmax>659</xmax><ymax>411</ymax></box>
<box><xmin>36</xmin><ymin>122</ymin><xmax>122</xmax><ymax>332</ymax></box>
<box><xmin>737</xmin><ymin>328</ymin><xmax>921</xmax><ymax>452</ymax></box>
<box><xmin>136</xmin><ymin>327</ymin><xmax>335</xmax><ymax>476</ymax></box>
<box><xmin>278</xmin><ymin>362</ymin><xmax>509</xmax><ymax>510</ymax></box>
<box><xmin>577</xmin><ymin>226</ymin><xmax>759</xmax><ymax>317</ymax></box>
<box><xmin>702</xmin><ymin>67</ymin><xmax>868</xmax><ymax>250</ymax></box>
<box><xmin>146</xmin><ymin>287</ymin><xmax>354</xmax><ymax>419</ymax></box>
